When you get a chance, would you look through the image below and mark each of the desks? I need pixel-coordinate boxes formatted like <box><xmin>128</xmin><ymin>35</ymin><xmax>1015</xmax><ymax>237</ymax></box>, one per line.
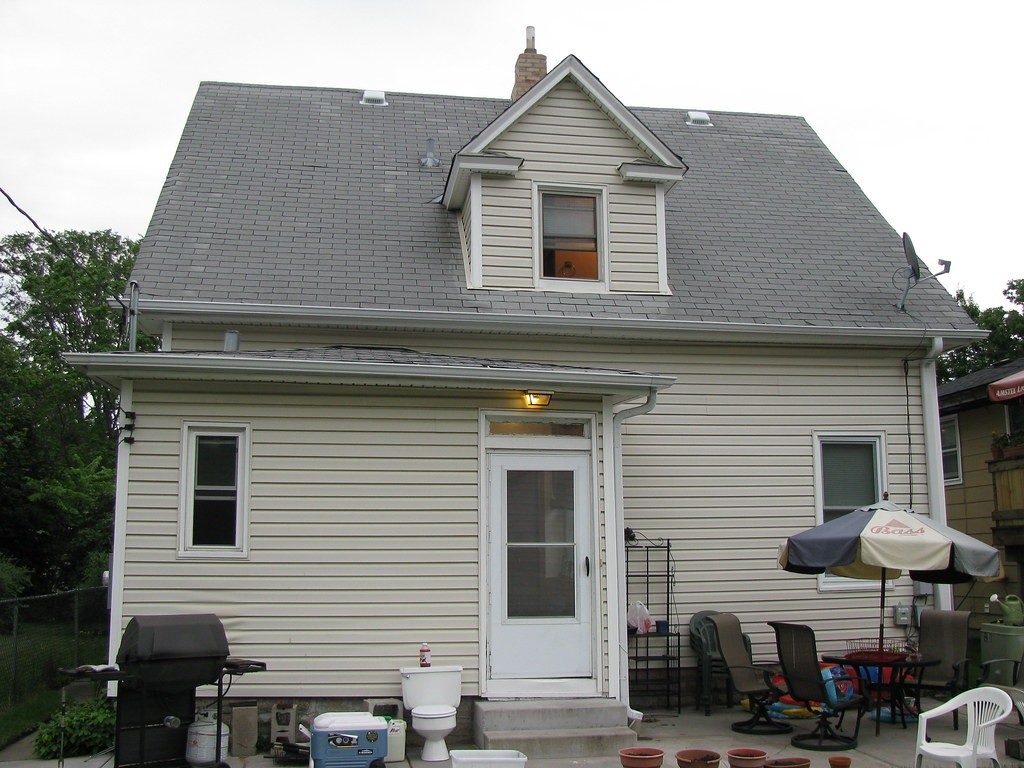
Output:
<box><xmin>54</xmin><ymin>661</ymin><xmax>267</xmax><ymax>768</ymax></box>
<box><xmin>822</xmin><ymin>652</ymin><xmax>941</xmax><ymax>743</ymax></box>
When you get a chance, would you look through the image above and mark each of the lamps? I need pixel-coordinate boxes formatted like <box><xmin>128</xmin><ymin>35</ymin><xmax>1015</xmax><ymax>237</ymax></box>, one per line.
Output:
<box><xmin>523</xmin><ymin>389</ymin><xmax>554</xmax><ymax>407</ymax></box>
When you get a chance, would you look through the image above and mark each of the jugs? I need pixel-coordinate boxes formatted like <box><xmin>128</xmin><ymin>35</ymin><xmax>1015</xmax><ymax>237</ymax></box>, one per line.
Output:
<box><xmin>990</xmin><ymin>594</ymin><xmax>1024</xmax><ymax>626</ymax></box>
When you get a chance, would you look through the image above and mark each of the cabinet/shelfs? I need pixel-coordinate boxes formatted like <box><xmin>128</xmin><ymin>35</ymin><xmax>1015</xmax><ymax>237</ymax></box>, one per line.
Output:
<box><xmin>625</xmin><ymin>528</ymin><xmax>682</xmax><ymax>715</ymax></box>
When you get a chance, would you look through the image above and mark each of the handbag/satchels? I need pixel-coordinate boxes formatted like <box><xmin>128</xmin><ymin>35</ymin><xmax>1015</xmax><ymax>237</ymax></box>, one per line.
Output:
<box><xmin>628</xmin><ymin>599</ymin><xmax>651</xmax><ymax>634</ymax></box>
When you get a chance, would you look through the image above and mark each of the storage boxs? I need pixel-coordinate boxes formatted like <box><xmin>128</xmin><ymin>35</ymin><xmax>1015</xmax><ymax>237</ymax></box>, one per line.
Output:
<box><xmin>308</xmin><ymin>712</ymin><xmax>388</xmax><ymax>768</ymax></box>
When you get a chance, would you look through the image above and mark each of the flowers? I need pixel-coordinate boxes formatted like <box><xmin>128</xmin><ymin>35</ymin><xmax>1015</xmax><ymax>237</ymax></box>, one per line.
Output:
<box><xmin>990</xmin><ymin>430</ymin><xmax>1024</xmax><ymax>449</ymax></box>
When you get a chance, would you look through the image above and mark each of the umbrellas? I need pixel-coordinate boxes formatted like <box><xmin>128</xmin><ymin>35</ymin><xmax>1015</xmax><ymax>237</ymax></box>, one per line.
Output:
<box><xmin>777</xmin><ymin>491</ymin><xmax>1005</xmax><ymax>736</ymax></box>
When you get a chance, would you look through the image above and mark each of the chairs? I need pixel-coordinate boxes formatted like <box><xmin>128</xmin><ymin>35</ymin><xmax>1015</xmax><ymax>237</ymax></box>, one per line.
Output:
<box><xmin>689</xmin><ymin>610</ymin><xmax>752</xmax><ymax>715</ymax></box>
<box><xmin>976</xmin><ymin>650</ymin><xmax>1024</xmax><ymax>725</ymax></box>
<box><xmin>767</xmin><ymin>622</ymin><xmax>870</xmax><ymax>752</ymax></box>
<box><xmin>914</xmin><ymin>686</ymin><xmax>1014</xmax><ymax>768</ymax></box>
<box><xmin>706</xmin><ymin>614</ymin><xmax>793</xmax><ymax>735</ymax></box>
<box><xmin>890</xmin><ymin>609</ymin><xmax>975</xmax><ymax>743</ymax></box>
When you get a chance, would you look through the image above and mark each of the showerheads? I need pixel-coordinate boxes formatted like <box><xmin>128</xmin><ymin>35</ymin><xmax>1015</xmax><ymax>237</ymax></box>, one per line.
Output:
<box><xmin>990</xmin><ymin>594</ymin><xmax>1024</xmax><ymax>626</ymax></box>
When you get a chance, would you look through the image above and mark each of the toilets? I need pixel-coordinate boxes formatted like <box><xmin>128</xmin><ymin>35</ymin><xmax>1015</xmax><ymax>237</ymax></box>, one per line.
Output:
<box><xmin>399</xmin><ymin>665</ymin><xmax>463</xmax><ymax>762</ymax></box>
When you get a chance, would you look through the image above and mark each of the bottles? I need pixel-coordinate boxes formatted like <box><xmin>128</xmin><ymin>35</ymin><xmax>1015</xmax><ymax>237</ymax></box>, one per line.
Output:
<box><xmin>420</xmin><ymin>643</ymin><xmax>431</xmax><ymax>666</ymax></box>
<box><xmin>912</xmin><ymin>642</ymin><xmax>919</xmax><ymax>658</ymax></box>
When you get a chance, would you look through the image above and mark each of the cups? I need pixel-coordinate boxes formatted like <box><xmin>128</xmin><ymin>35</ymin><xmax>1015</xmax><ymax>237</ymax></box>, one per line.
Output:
<box><xmin>655</xmin><ymin>620</ymin><xmax>669</xmax><ymax>634</ymax></box>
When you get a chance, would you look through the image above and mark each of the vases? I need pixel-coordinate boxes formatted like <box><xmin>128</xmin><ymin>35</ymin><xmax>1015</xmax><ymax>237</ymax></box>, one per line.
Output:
<box><xmin>829</xmin><ymin>757</ymin><xmax>852</xmax><ymax>768</ymax></box>
<box><xmin>675</xmin><ymin>749</ymin><xmax>722</xmax><ymax>768</ymax></box>
<box><xmin>765</xmin><ymin>758</ymin><xmax>811</xmax><ymax>768</ymax></box>
<box><xmin>618</xmin><ymin>747</ymin><xmax>666</xmax><ymax>768</ymax></box>
<box><xmin>991</xmin><ymin>444</ymin><xmax>1024</xmax><ymax>460</ymax></box>
<box><xmin>726</xmin><ymin>749</ymin><xmax>768</xmax><ymax>768</ymax></box>
